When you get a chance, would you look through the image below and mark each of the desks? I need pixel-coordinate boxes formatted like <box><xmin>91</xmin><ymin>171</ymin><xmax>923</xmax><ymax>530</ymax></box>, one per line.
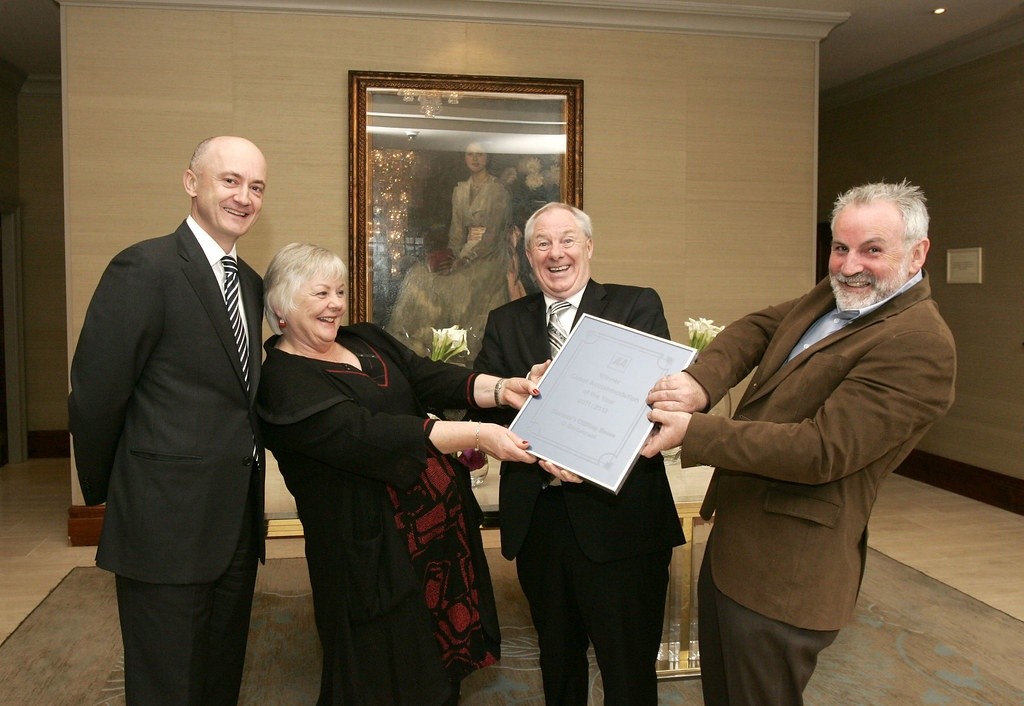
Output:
<box><xmin>258</xmin><ymin>450</ymin><xmax>737</xmax><ymax>684</ymax></box>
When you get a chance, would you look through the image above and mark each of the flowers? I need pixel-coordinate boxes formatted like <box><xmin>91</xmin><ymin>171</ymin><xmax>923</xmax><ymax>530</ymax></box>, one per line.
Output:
<box><xmin>682</xmin><ymin>317</ymin><xmax>726</xmax><ymax>361</ymax></box>
<box><xmin>426</xmin><ymin>323</ymin><xmax>472</xmax><ymax>363</ymax></box>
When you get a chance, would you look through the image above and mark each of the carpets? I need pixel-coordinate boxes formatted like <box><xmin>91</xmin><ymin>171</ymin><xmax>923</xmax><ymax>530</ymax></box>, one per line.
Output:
<box><xmin>0</xmin><ymin>516</ymin><xmax>1017</xmax><ymax>703</ymax></box>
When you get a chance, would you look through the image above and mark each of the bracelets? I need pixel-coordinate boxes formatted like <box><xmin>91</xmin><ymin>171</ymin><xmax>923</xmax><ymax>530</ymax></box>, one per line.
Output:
<box><xmin>474</xmin><ymin>421</ymin><xmax>481</xmax><ymax>452</ymax></box>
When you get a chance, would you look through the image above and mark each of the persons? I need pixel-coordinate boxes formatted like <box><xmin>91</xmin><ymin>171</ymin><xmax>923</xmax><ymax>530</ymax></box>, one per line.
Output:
<box><xmin>260</xmin><ymin>243</ymin><xmax>540</xmax><ymax>706</ymax></box>
<box><xmin>411</xmin><ymin>139</ymin><xmax>512</xmax><ymax>363</ymax></box>
<box><xmin>67</xmin><ymin>136</ymin><xmax>266</xmax><ymax>706</ymax></box>
<box><xmin>645</xmin><ymin>180</ymin><xmax>958</xmax><ymax>704</ymax></box>
<box><xmin>473</xmin><ymin>201</ymin><xmax>689</xmax><ymax>706</ymax></box>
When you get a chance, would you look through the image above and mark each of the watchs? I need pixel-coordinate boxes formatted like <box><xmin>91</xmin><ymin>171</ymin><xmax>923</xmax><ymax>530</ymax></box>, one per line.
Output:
<box><xmin>494</xmin><ymin>377</ymin><xmax>507</xmax><ymax>409</ymax></box>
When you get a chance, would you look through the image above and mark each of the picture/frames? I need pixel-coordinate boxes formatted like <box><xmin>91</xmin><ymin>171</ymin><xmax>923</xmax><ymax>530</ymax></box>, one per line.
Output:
<box><xmin>345</xmin><ymin>68</ymin><xmax>586</xmax><ymax>369</ymax></box>
<box><xmin>508</xmin><ymin>312</ymin><xmax>700</xmax><ymax>495</ymax></box>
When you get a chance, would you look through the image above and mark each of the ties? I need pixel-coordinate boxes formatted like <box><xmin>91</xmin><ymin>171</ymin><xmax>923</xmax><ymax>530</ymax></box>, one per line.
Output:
<box><xmin>222</xmin><ymin>255</ymin><xmax>252</xmax><ymax>392</ymax></box>
<box><xmin>547</xmin><ymin>302</ymin><xmax>574</xmax><ymax>360</ymax></box>
